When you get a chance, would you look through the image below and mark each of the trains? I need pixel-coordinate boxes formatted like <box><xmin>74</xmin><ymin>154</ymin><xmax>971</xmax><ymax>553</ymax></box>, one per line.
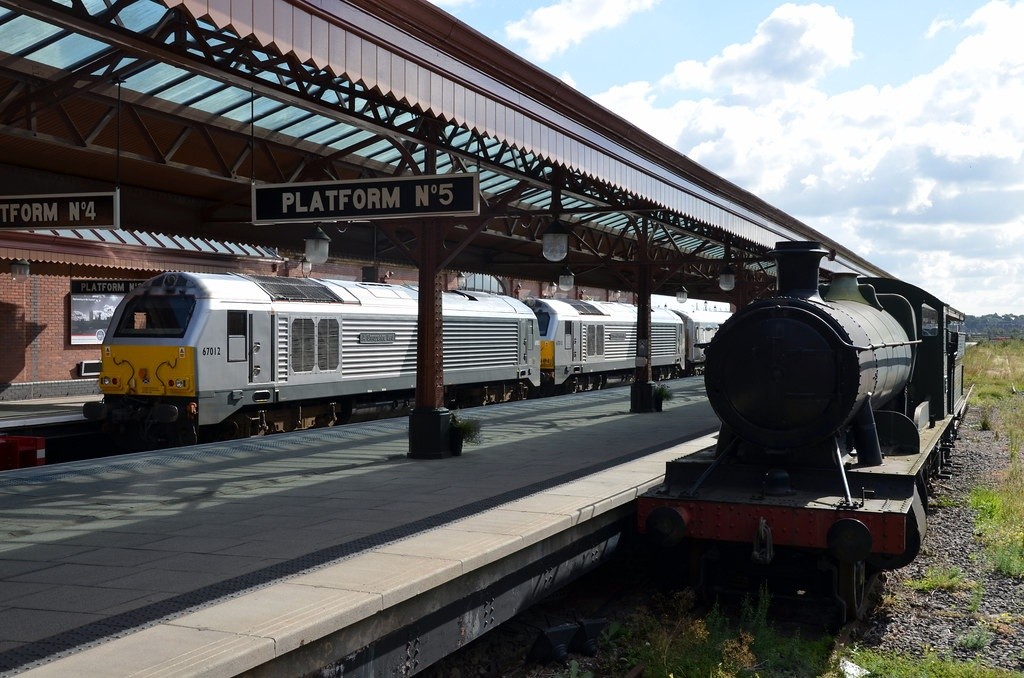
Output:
<box><xmin>634</xmin><ymin>237</ymin><xmax>969</xmax><ymax>637</ymax></box>
<box><xmin>82</xmin><ymin>270</ymin><xmax>736</xmax><ymax>452</ymax></box>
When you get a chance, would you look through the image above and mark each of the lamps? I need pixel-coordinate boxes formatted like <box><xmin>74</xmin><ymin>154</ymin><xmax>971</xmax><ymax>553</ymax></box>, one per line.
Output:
<box><xmin>249</xmin><ymin>100</ymin><xmax>740</xmax><ymax>303</ymax></box>
